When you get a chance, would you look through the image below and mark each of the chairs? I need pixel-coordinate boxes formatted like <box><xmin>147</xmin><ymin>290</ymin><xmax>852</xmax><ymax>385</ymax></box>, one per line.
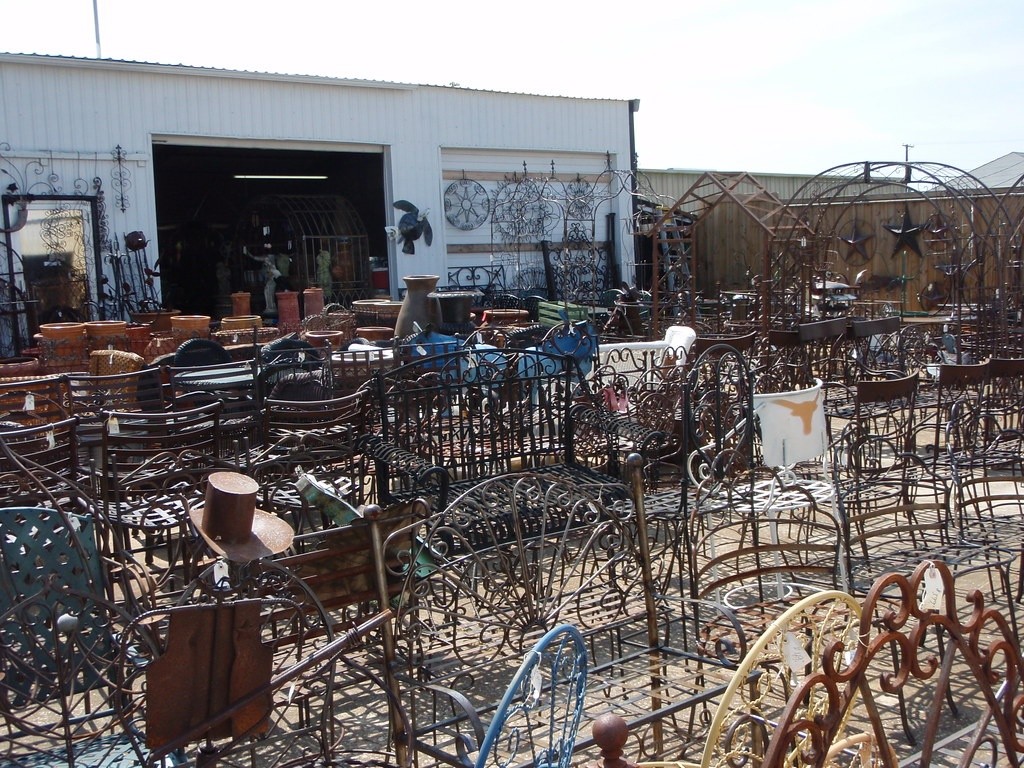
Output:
<box><xmin>0</xmin><ymin>288</ymin><xmax>1024</xmax><ymax>768</ymax></box>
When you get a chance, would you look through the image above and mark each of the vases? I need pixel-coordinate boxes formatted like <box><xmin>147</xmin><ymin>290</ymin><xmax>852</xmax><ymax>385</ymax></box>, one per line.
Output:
<box><xmin>0</xmin><ymin>270</ymin><xmax>720</xmax><ymax>468</ymax></box>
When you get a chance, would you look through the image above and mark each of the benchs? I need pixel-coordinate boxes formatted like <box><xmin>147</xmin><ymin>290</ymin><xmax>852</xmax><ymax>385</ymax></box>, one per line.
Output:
<box><xmin>364</xmin><ymin>453</ymin><xmax>763</xmax><ymax>768</ymax></box>
<box><xmin>355</xmin><ymin>350</ymin><xmax>662</xmax><ymax>624</ymax></box>
